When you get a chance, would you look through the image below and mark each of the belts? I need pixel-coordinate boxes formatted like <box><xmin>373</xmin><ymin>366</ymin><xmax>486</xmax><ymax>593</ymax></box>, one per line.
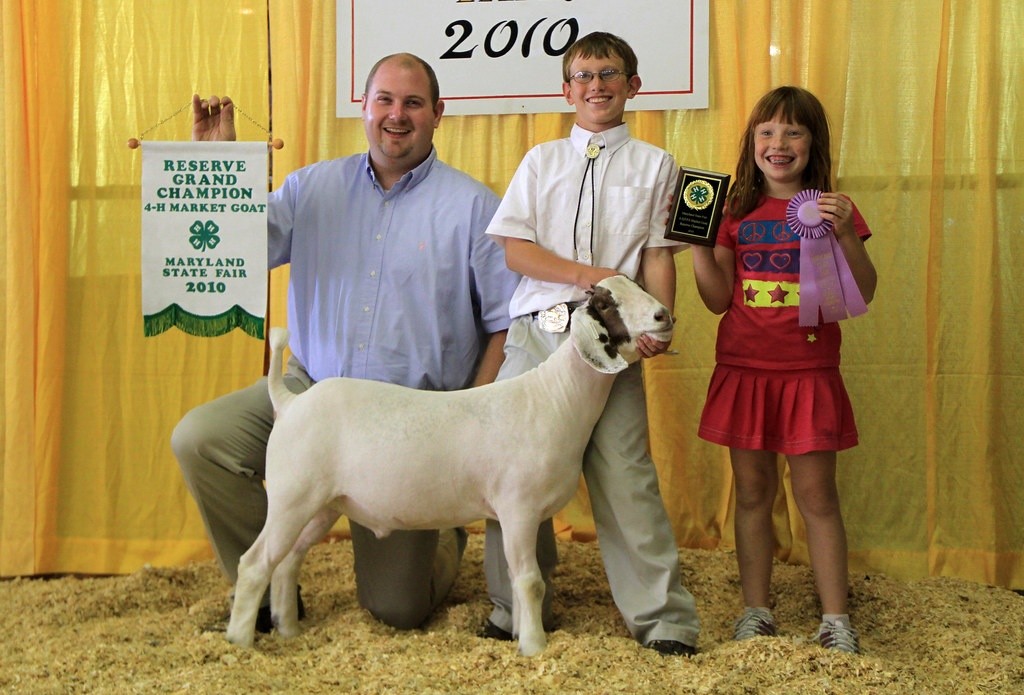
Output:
<box><xmin>532</xmin><ymin>302</ymin><xmax>579</xmax><ymax>332</ymax></box>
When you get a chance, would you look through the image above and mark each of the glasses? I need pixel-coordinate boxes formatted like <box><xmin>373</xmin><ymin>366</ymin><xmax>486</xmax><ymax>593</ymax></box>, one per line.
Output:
<box><xmin>570</xmin><ymin>69</ymin><xmax>629</xmax><ymax>84</ymax></box>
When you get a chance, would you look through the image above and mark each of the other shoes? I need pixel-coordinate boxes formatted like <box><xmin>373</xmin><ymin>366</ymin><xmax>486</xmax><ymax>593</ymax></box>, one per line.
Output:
<box><xmin>475</xmin><ymin>615</ymin><xmax>554</xmax><ymax>641</ymax></box>
<box><xmin>203</xmin><ymin>586</ymin><xmax>303</xmax><ymax>633</ymax></box>
<box><xmin>648</xmin><ymin>640</ymin><xmax>695</xmax><ymax>659</ymax></box>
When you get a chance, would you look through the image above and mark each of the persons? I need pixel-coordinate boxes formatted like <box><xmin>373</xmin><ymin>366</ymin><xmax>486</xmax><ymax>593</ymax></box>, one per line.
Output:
<box><xmin>692</xmin><ymin>85</ymin><xmax>877</xmax><ymax>656</ymax></box>
<box><xmin>171</xmin><ymin>52</ymin><xmax>522</xmax><ymax>629</ymax></box>
<box><xmin>484</xmin><ymin>33</ymin><xmax>700</xmax><ymax>657</ymax></box>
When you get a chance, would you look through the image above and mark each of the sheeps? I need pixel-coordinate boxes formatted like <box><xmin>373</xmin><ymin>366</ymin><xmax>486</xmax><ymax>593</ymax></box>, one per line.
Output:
<box><xmin>224</xmin><ymin>276</ymin><xmax>677</xmax><ymax>658</ymax></box>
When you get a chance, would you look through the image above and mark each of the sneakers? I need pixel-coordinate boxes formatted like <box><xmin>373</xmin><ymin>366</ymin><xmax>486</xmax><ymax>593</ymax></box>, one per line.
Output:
<box><xmin>819</xmin><ymin>621</ymin><xmax>860</xmax><ymax>655</ymax></box>
<box><xmin>733</xmin><ymin>608</ymin><xmax>776</xmax><ymax>641</ymax></box>
